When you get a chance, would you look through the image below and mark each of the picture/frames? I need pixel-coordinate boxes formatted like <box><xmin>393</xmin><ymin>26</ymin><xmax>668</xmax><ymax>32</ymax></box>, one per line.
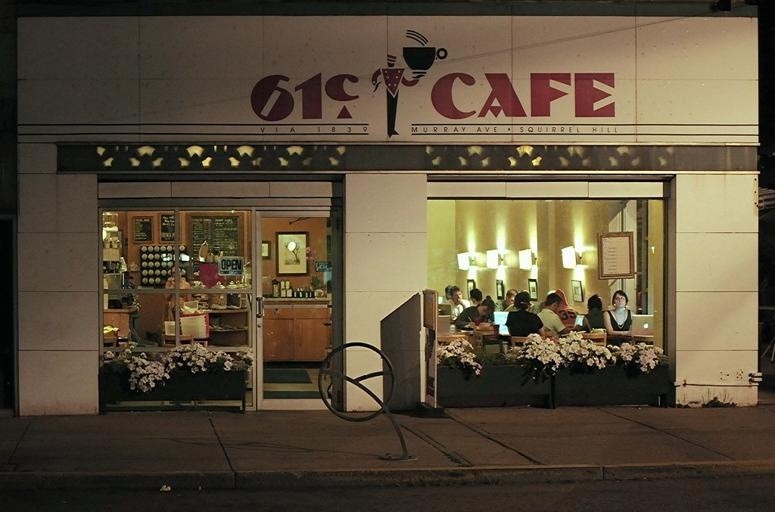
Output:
<box><xmin>496</xmin><ymin>280</ymin><xmax>504</xmax><ymax>299</ymax></box>
<box><xmin>528</xmin><ymin>279</ymin><xmax>538</xmax><ymax>301</ymax></box>
<box><xmin>467</xmin><ymin>279</ymin><xmax>475</xmax><ymax>298</ymax></box>
<box><xmin>275</xmin><ymin>231</ymin><xmax>310</xmax><ymax>276</ymax></box>
<box><xmin>261</xmin><ymin>240</ymin><xmax>271</xmax><ymax>260</ymax></box>
<box><xmin>571</xmin><ymin>280</ymin><xmax>583</xmax><ymax>302</ymax></box>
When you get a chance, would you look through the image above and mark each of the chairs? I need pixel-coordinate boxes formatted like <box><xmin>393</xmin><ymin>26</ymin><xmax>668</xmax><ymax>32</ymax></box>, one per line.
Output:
<box><xmin>436</xmin><ymin>323</ymin><xmax>653</xmax><ymax>353</ymax></box>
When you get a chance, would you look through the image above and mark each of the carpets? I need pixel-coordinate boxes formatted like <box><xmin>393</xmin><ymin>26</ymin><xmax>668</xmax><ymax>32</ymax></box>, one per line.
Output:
<box><xmin>263</xmin><ymin>370</ymin><xmax>312</xmax><ymax>383</ymax></box>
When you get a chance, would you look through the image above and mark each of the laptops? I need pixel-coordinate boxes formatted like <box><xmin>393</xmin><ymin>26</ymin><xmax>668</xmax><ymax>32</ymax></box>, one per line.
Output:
<box><xmin>632</xmin><ymin>315</ymin><xmax>655</xmax><ymax>336</ymax></box>
<box><xmin>573</xmin><ymin>315</ymin><xmax>584</xmax><ymax>327</ymax></box>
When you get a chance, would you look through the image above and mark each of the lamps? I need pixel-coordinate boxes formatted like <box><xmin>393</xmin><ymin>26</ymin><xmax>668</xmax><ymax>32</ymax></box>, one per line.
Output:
<box><xmin>487</xmin><ymin>248</ymin><xmax>502</xmax><ymax>269</ymax></box>
<box><xmin>519</xmin><ymin>249</ymin><xmax>537</xmax><ymax>271</ymax></box>
<box><xmin>561</xmin><ymin>246</ymin><xmax>581</xmax><ymax>269</ymax></box>
<box><xmin>456</xmin><ymin>251</ymin><xmax>473</xmax><ymax>271</ymax></box>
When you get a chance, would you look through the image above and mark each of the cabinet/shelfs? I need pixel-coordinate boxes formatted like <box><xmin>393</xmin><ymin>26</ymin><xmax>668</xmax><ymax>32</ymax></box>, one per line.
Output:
<box><xmin>179</xmin><ymin>294</ymin><xmax>251</xmax><ymax>348</ymax></box>
<box><xmin>262</xmin><ymin>297</ymin><xmax>330</xmax><ymax>361</ymax></box>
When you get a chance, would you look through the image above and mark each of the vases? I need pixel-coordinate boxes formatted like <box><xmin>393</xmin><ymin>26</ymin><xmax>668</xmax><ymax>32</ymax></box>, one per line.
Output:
<box><xmin>437</xmin><ymin>363</ymin><xmax>672</xmax><ymax>408</ymax></box>
<box><xmin>102</xmin><ymin>361</ymin><xmax>246</xmax><ymax>416</ymax></box>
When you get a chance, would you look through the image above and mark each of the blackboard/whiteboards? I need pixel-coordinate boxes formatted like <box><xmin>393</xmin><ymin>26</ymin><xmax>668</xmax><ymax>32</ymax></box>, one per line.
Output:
<box><xmin>158</xmin><ymin>212</ymin><xmax>183</xmax><ymax>244</ymax></box>
<box><xmin>184</xmin><ymin>211</ymin><xmax>244</xmax><ymax>281</ymax></box>
<box><xmin>131</xmin><ymin>215</ymin><xmax>154</xmax><ymax>244</ymax></box>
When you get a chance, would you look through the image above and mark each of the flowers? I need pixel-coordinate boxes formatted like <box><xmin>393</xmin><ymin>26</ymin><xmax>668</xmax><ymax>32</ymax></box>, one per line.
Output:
<box><xmin>437</xmin><ymin>333</ymin><xmax>665</xmax><ymax>380</ymax></box>
<box><xmin>104</xmin><ymin>340</ymin><xmax>251</xmax><ymax>392</ymax></box>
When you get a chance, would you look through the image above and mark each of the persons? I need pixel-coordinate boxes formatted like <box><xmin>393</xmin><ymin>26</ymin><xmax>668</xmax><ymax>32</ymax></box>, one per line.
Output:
<box><xmin>442</xmin><ymin>285</ymin><xmax>634</xmax><ymax>347</ymax></box>
<box><xmin>164</xmin><ymin>263</ymin><xmax>191</xmax><ymax>320</ymax></box>
<box><xmin>211</xmin><ymin>249</ymin><xmax>224</xmax><ymax>275</ymax></box>
